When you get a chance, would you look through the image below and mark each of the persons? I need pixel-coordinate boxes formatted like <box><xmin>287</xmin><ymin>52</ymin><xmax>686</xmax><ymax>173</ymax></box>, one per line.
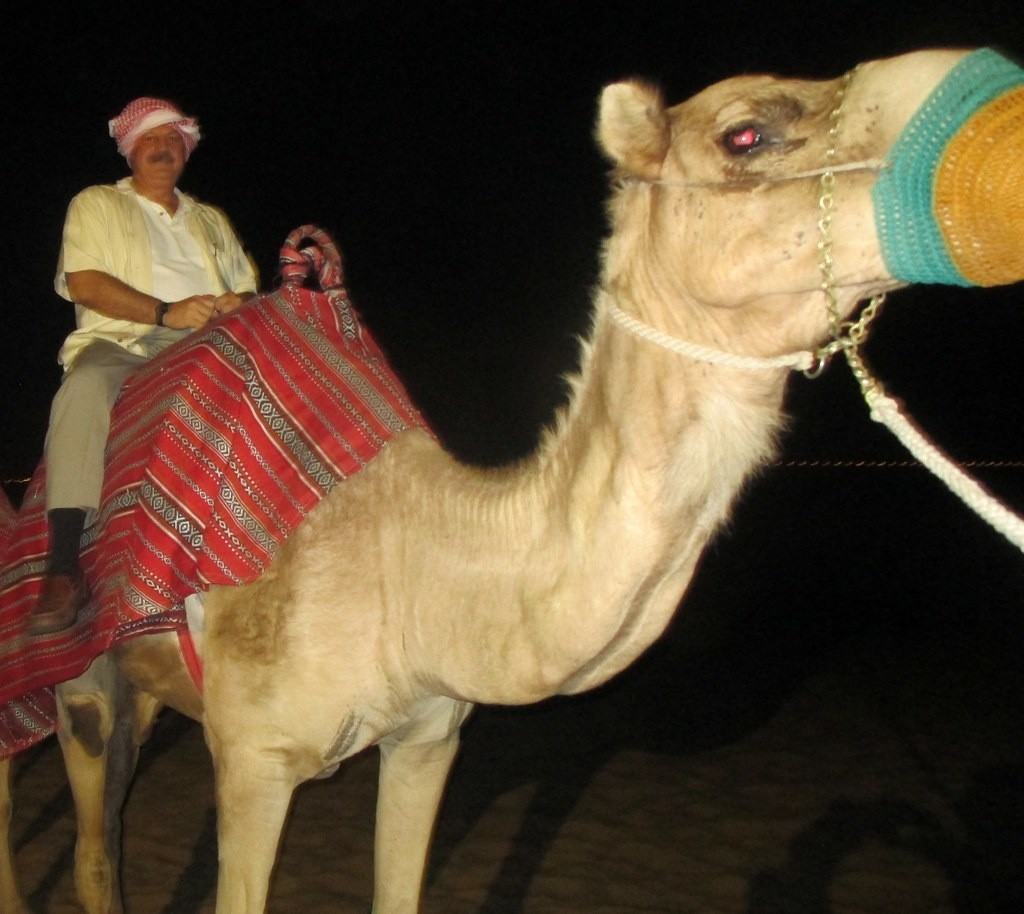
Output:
<box><xmin>22</xmin><ymin>96</ymin><xmax>260</xmax><ymax>639</ymax></box>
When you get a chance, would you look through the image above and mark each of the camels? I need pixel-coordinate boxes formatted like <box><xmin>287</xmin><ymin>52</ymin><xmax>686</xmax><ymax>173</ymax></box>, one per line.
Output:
<box><xmin>2</xmin><ymin>48</ymin><xmax>1024</xmax><ymax>914</ymax></box>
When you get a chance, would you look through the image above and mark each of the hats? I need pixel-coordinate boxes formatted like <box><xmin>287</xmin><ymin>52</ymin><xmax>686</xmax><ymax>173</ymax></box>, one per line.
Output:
<box><xmin>109</xmin><ymin>97</ymin><xmax>200</xmax><ymax>169</ymax></box>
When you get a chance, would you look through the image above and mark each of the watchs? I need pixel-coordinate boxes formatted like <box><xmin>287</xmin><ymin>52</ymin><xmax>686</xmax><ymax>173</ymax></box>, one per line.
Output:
<box><xmin>153</xmin><ymin>299</ymin><xmax>173</xmax><ymax>329</ymax></box>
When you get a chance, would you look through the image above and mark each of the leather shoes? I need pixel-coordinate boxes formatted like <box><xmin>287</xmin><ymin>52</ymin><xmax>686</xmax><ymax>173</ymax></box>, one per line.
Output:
<box><xmin>23</xmin><ymin>571</ymin><xmax>92</xmax><ymax>635</ymax></box>
